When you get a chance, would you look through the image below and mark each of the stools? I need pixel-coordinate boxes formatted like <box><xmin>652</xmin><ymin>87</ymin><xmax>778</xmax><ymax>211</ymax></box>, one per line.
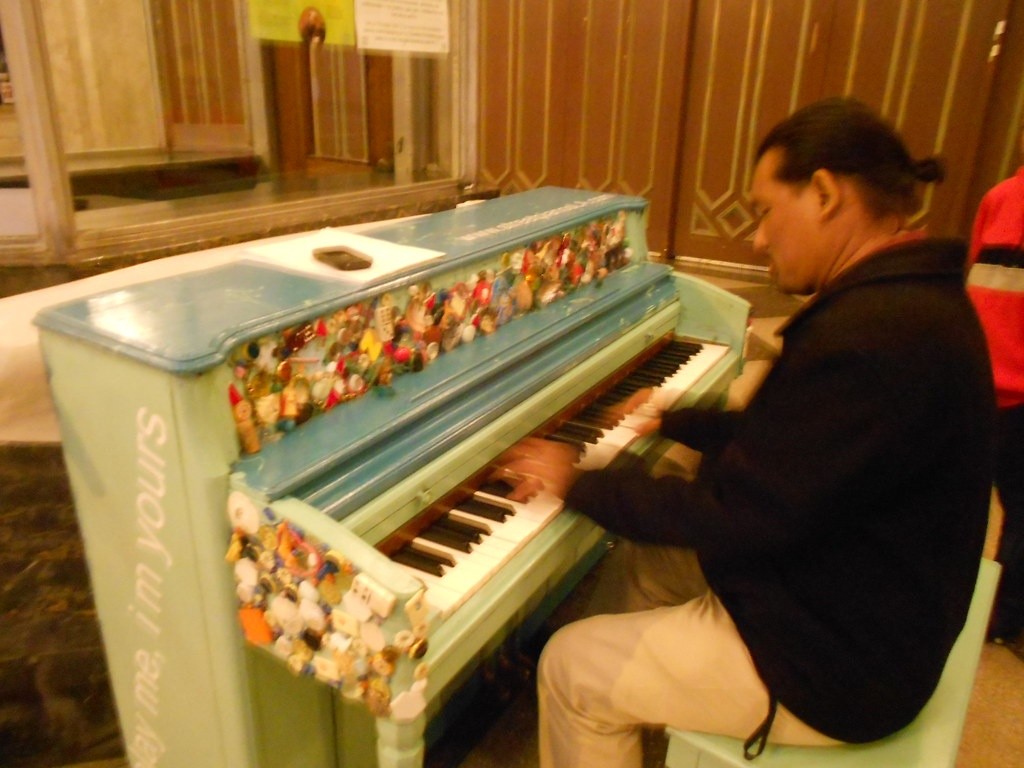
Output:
<box><xmin>664</xmin><ymin>558</ymin><xmax>1005</xmax><ymax>768</ymax></box>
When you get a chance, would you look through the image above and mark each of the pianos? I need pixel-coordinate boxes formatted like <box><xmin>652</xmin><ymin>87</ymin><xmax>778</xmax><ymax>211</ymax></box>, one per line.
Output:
<box><xmin>28</xmin><ymin>179</ymin><xmax>753</xmax><ymax>768</ymax></box>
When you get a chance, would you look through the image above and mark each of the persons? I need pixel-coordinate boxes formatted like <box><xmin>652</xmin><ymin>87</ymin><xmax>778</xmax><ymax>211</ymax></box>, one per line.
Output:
<box><xmin>965</xmin><ymin>161</ymin><xmax>1024</xmax><ymax>642</ymax></box>
<box><xmin>495</xmin><ymin>100</ymin><xmax>998</xmax><ymax>768</ymax></box>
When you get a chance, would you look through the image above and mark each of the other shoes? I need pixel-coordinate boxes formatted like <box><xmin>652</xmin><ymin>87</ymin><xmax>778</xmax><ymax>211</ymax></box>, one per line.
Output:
<box><xmin>990</xmin><ymin>630</ymin><xmax>1024</xmax><ymax>662</ymax></box>
